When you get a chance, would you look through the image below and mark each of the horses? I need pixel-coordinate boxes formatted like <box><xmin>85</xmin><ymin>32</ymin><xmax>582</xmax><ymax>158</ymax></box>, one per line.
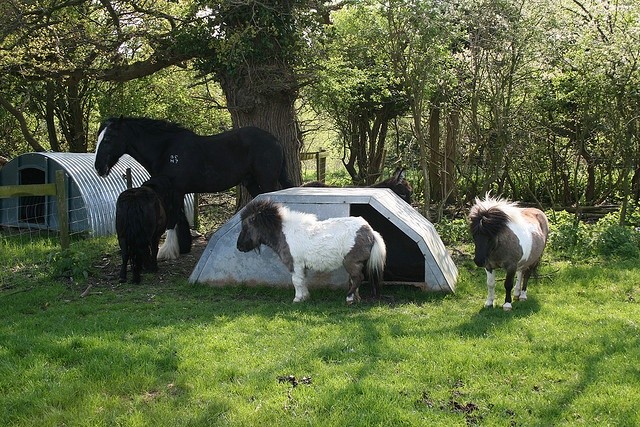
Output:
<box><xmin>466</xmin><ymin>188</ymin><xmax>550</xmax><ymax>314</ymax></box>
<box><xmin>113</xmin><ymin>175</ymin><xmax>171</xmax><ymax>288</ymax></box>
<box><xmin>234</xmin><ymin>198</ymin><xmax>388</xmax><ymax>307</ymax></box>
<box><xmin>92</xmin><ymin>114</ymin><xmax>293</xmax><ymax>263</ymax></box>
<box><xmin>299</xmin><ymin>172</ymin><xmax>415</xmax><ymax>207</ymax></box>
